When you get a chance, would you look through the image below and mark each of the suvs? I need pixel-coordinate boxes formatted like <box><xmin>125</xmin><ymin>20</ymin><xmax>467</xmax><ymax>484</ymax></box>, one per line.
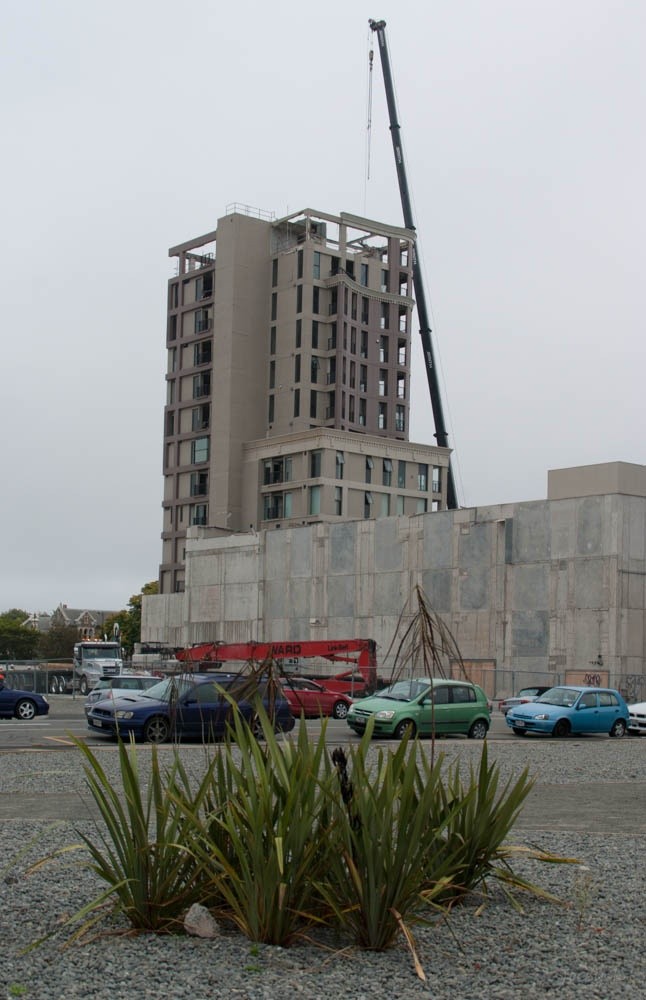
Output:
<box><xmin>83</xmin><ymin>674</ymin><xmax>166</xmax><ymax>716</ymax></box>
<box><xmin>86</xmin><ymin>672</ymin><xmax>296</xmax><ymax>745</ymax></box>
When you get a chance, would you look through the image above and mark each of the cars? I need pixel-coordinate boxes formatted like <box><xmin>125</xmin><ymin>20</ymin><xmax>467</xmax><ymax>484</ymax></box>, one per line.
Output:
<box><xmin>505</xmin><ymin>685</ymin><xmax>631</xmax><ymax>738</ymax></box>
<box><xmin>0</xmin><ymin>681</ymin><xmax>51</xmax><ymax>720</ymax></box>
<box><xmin>626</xmin><ymin>701</ymin><xmax>646</xmax><ymax>735</ymax></box>
<box><xmin>277</xmin><ymin>677</ymin><xmax>354</xmax><ymax>720</ymax></box>
<box><xmin>498</xmin><ymin>684</ymin><xmax>578</xmax><ymax>717</ymax></box>
<box><xmin>346</xmin><ymin>677</ymin><xmax>494</xmax><ymax>741</ymax></box>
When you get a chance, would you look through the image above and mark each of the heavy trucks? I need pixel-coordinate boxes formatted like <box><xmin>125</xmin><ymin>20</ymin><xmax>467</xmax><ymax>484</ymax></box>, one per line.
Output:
<box><xmin>0</xmin><ymin>640</ymin><xmax>125</xmax><ymax>696</ymax></box>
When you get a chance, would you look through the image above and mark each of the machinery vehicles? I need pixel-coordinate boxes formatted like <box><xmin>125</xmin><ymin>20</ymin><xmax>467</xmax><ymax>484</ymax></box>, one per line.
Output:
<box><xmin>358</xmin><ymin>16</ymin><xmax>459</xmax><ymax>511</ymax></box>
<box><xmin>173</xmin><ymin>637</ymin><xmax>404</xmax><ymax>697</ymax></box>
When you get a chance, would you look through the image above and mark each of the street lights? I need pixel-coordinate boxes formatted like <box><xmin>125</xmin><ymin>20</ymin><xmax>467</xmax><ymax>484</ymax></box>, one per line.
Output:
<box><xmin>96</xmin><ymin>611</ymin><xmax>105</xmax><ymax>640</ymax></box>
